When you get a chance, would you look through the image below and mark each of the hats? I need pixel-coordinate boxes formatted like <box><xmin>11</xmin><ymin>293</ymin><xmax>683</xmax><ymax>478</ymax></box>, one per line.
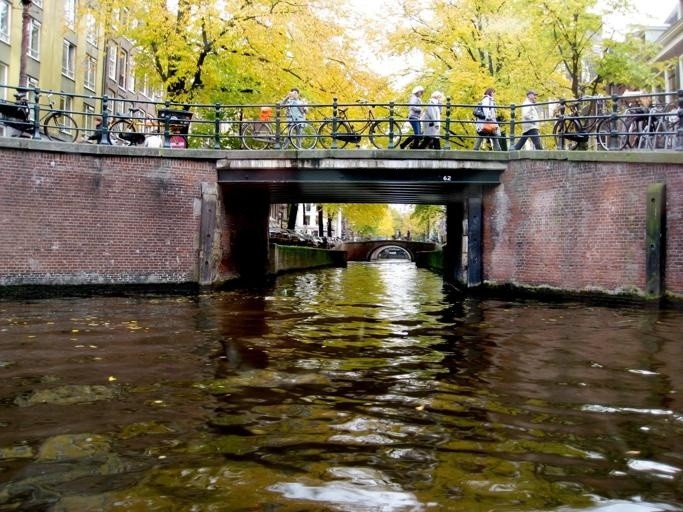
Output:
<box><xmin>412</xmin><ymin>86</ymin><xmax>425</xmax><ymax>94</ymax></box>
<box><xmin>527</xmin><ymin>90</ymin><xmax>538</xmax><ymax>96</ymax></box>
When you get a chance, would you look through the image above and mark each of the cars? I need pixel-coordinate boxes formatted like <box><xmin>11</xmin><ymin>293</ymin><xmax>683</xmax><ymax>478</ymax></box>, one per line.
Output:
<box><xmin>268</xmin><ymin>226</ymin><xmax>338</xmax><ymax>249</ymax></box>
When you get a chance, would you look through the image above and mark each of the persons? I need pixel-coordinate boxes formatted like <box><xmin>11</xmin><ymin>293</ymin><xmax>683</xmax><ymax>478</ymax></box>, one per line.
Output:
<box><xmin>279</xmin><ymin>88</ymin><xmax>308</xmax><ymax>148</ymax></box>
<box><xmin>406</xmin><ymin>230</ymin><xmax>410</xmax><ymax>240</ymax></box>
<box><xmin>513</xmin><ymin>90</ymin><xmax>544</xmax><ymax>150</ymax></box>
<box><xmin>395</xmin><ymin>229</ymin><xmax>401</xmax><ymax>240</ymax></box>
<box><xmin>143</xmin><ymin>130</ymin><xmax>162</xmax><ymax>149</ymax></box>
<box><xmin>418</xmin><ymin>91</ymin><xmax>445</xmax><ymax>149</ymax></box>
<box><xmin>473</xmin><ymin>87</ymin><xmax>502</xmax><ymax>151</ymax></box>
<box><xmin>399</xmin><ymin>86</ymin><xmax>426</xmax><ymax>150</ymax></box>
<box><xmin>80</xmin><ymin>116</ymin><xmax>103</xmax><ymax>144</ymax></box>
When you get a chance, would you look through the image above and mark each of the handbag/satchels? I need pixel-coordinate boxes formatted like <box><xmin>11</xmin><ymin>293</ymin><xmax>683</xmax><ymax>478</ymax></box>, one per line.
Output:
<box><xmin>477</xmin><ymin>121</ymin><xmax>499</xmax><ymax>136</ymax></box>
<box><xmin>473</xmin><ymin>102</ymin><xmax>486</xmax><ymax>120</ymax></box>
